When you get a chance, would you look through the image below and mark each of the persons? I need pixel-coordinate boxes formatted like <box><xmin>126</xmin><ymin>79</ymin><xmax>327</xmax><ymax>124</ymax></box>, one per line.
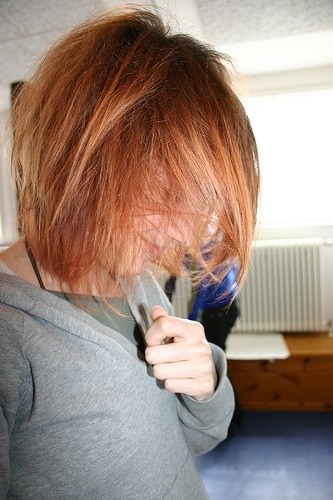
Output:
<box><xmin>185</xmin><ymin>234</ymin><xmax>239</xmax><ymax>352</ymax></box>
<box><xmin>0</xmin><ymin>4</ymin><xmax>261</xmax><ymax>500</ymax></box>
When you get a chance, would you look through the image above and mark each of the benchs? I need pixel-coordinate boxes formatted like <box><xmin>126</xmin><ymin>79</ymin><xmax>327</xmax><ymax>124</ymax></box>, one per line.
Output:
<box><xmin>229</xmin><ymin>334</ymin><xmax>332</xmax><ymax>415</ymax></box>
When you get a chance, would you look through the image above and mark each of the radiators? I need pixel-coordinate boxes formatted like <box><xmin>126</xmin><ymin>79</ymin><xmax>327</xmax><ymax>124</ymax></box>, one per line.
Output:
<box><xmin>209</xmin><ymin>238</ymin><xmax>333</xmax><ymax>333</ymax></box>
